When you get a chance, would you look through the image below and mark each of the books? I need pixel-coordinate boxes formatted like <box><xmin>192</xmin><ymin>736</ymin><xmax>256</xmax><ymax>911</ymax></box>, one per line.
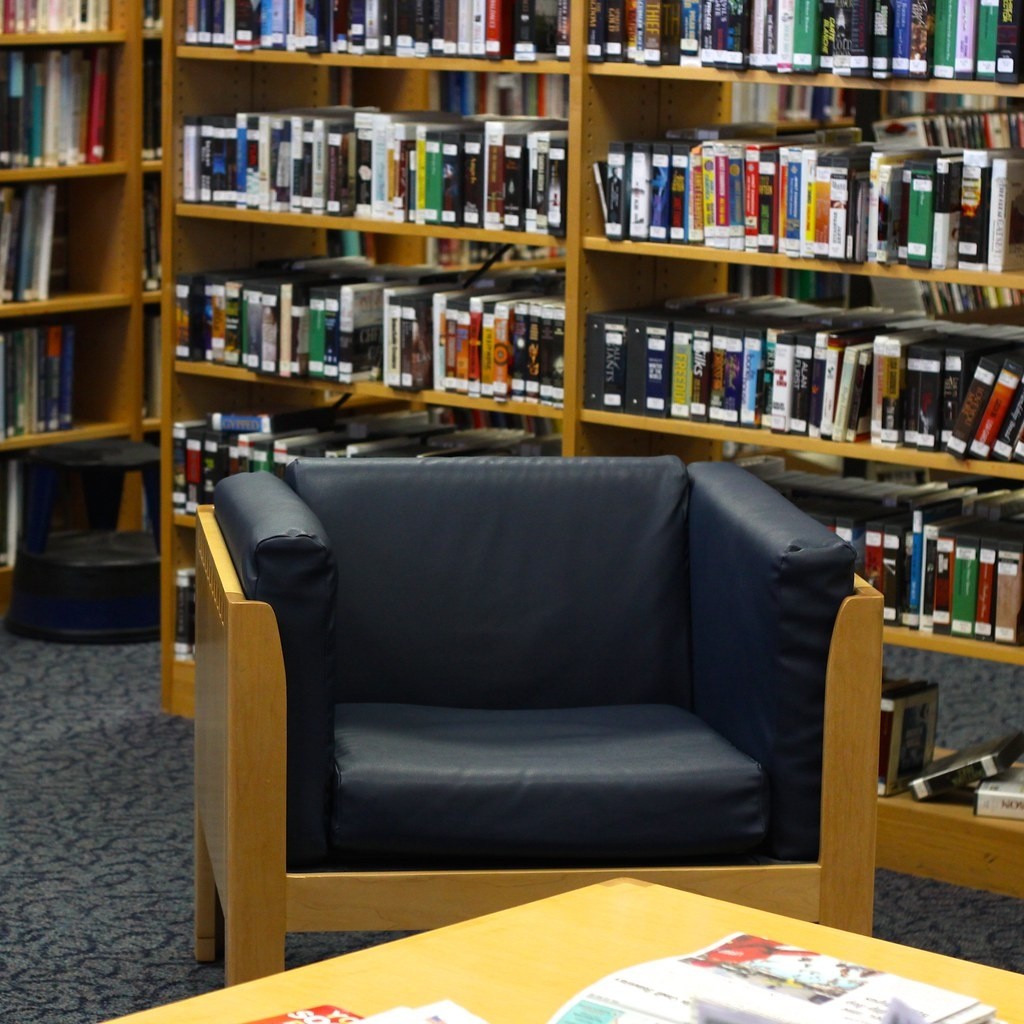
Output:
<box><xmin>243</xmin><ymin>932</ymin><xmax>1014</xmax><ymax>1024</ymax></box>
<box><xmin>0</xmin><ymin>0</ymin><xmax>1024</xmax><ymax>817</ymax></box>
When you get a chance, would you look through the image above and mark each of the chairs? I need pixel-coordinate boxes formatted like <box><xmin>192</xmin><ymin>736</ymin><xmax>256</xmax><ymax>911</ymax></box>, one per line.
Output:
<box><xmin>193</xmin><ymin>456</ymin><xmax>883</xmax><ymax>990</ymax></box>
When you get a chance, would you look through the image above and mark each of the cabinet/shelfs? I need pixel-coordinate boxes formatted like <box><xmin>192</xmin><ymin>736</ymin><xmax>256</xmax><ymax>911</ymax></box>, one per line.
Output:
<box><xmin>0</xmin><ymin>0</ymin><xmax>162</xmax><ymax>626</ymax></box>
<box><xmin>162</xmin><ymin>0</ymin><xmax>1024</xmax><ymax>902</ymax></box>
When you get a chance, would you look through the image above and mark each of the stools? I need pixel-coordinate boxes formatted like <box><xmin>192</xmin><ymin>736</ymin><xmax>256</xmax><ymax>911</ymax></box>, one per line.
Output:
<box><xmin>5</xmin><ymin>438</ymin><xmax>161</xmax><ymax>642</ymax></box>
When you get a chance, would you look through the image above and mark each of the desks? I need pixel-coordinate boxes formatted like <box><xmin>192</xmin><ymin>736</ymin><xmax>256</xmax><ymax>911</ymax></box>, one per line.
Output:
<box><xmin>95</xmin><ymin>874</ymin><xmax>1024</xmax><ymax>1024</ymax></box>
